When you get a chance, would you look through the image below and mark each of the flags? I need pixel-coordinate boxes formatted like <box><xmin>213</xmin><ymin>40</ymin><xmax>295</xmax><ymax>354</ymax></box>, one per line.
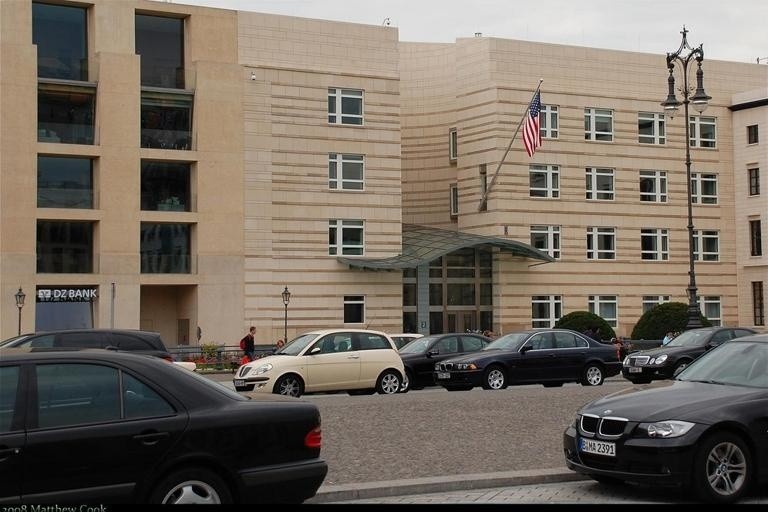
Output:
<box><xmin>521</xmin><ymin>89</ymin><xmax>542</xmax><ymax>159</ymax></box>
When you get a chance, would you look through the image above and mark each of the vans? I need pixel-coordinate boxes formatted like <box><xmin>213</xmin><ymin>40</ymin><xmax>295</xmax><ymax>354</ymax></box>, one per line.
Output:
<box><xmin>0</xmin><ymin>347</ymin><xmax>328</xmax><ymax>504</ymax></box>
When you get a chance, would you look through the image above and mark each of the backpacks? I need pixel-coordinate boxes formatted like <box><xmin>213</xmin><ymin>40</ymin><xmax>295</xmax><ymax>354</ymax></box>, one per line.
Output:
<box><xmin>240</xmin><ymin>334</ymin><xmax>251</xmax><ymax>349</ymax></box>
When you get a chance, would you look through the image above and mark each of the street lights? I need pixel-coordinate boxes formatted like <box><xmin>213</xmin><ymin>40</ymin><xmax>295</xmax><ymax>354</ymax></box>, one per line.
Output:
<box><xmin>13</xmin><ymin>288</ymin><xmax>26</xmax><ymax>336</ymax></box>
<box><xmin>282</xmin><ymin>286</ymin><xmax>291</xmax><ymax>345</ymax></box>
<box><xmin>661</xmin><ymin>28</ymin><xmax>712</xmax><ymax>329</ymax></box>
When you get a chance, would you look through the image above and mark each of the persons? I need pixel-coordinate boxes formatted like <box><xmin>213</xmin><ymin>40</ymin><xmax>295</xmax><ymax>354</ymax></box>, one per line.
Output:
<box><xmin>277</xmin><ymin>340</ymin><xmax>284</xmax><ymax>349</ymax></box>
<box><xmin>240</xmin><ymin>327</ymin><xmax>257</xmax><ymax>361</ymax></box>
<box><xmin>591</xmin><ymin>326</ymin><xmax>602</xmax><ymax>343</ymax></box>
<box><xmin>662</xmin><ymin>331</ymin><xmax>675</xmax><ymax>345</ymax></box>
<box><xmin>482</xmin><ymin>330</ymin><xmax>497</xmax><ymax>339</ymax></box>
<box><xmin>611</xmin><ymin>336</ymin><xmax>635</xmax><ymax>363</ymax></box>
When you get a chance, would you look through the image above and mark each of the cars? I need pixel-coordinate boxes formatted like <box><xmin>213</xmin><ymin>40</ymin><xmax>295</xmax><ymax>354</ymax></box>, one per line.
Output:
<box><xmin>1</xmin><ymin>328</ymin><xmax>175</xmax><ymax>402</ymax></box>
<box><xmin>232</xmin><ymin>327</ymin><xmax>408</xmax><ymax>397</ymax></box>
<box><xmin>334</xmin><ymin>333</ymin><xmax>424</xmax><ymax>351</ymax></box>
<box><xmin>562</xmin><ymin>335</ymin><xmax>767</xmax><ymax>504</ymax></box>
<box><xmin>392</xmin><ymin>333</ymin><xmax>492</xmax><ymax>391</ymax></box>
<box><xmin>623</xmin><ymin>327</ymin><xmax>765</xmax><ymax>384</ymax></box>
<box><xmin>434</xmin><ymin>327</ymin><xmax>623</xmax><ymax>391</ymax></box>
